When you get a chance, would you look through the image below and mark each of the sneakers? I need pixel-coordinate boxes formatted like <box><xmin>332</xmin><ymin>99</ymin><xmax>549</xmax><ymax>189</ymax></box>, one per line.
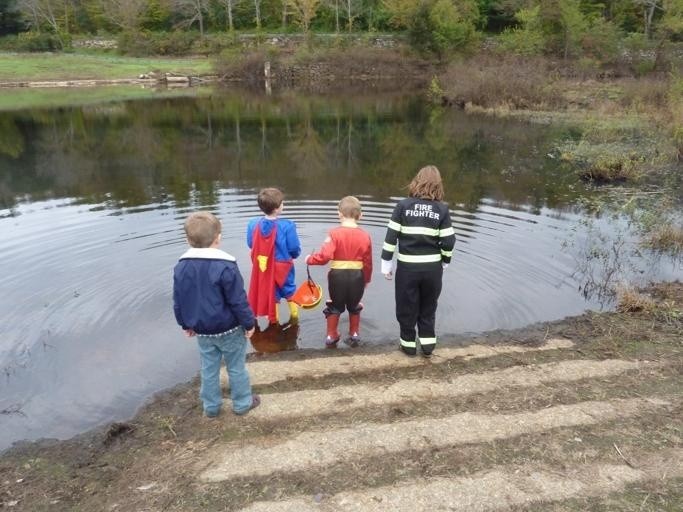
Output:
<box><xmin>423</xmin><ymin>350</ymin><xmax>432</xmax><ymax>357</ymax></box>
<box><xmin>350</xmin><ymin>335</ymin><xmax>360</xmax><ymax>342</ymax></box>
<box><xmin>249</xmin><ymin>396</ymin><xmax>260</xmax><ymax>409</ymax></box>
<box><xmin>399</xmin><ymin>342</ymin><xmax>416</xmax><ymax>355</ymax></box>
<box><xmin>327</xmin><ymin>335</ymin><xmax>340</xmax><ymax>344</ymax></box>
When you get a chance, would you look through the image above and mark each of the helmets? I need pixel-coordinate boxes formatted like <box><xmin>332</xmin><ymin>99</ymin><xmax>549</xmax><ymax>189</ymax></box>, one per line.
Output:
<box><xmin>288</xmin><ymin>280</ymin><xmax>322</xmax><ymax>308</ymax></box>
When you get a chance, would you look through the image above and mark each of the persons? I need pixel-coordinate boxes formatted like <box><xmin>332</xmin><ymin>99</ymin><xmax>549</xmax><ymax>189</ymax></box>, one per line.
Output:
<box><xmin>246</xmin><ymin>187</ymin><xmax>302</xmax><ymax>328</ymax></box>
<box><xmin>305</xmin><ymin>194</ymin><xmax>374</xmax><ymax>346</ymax></box>
<box><xmin>379</xmin><ymin>164</ymin><xmax>456</xmax><ymax>357</ymax></box>
<box><xmin>171</xmin><ymin>210</ymin><xmax>262</xmax><ymax>419</ymax></box>
